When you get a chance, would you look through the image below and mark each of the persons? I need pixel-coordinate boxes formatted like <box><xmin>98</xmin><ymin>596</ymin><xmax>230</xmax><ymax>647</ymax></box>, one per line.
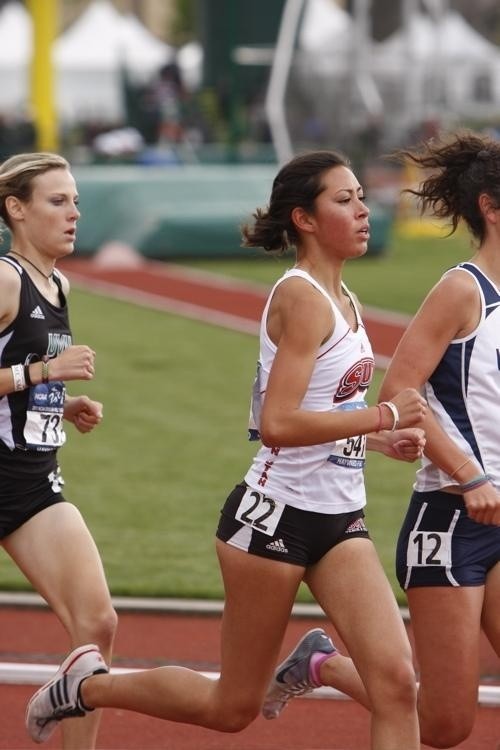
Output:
<box><xmin>0</xmin><ymin>151</ymin><xmax>120</xmax><ymax>748</ymax></box>
<box><xmin>258</xmin><ymin>133</ymin><xmax>499</xmax><ymax>748</ymax></box>
<box><xmin>23</xmin><ymin>146</ymin><xmax>431</xmax><ymax>748</ymax></box>
<box><xmin>156</xmin><ymin>61</ymin><xmax>221</xmax><ymax>146</ymax></box>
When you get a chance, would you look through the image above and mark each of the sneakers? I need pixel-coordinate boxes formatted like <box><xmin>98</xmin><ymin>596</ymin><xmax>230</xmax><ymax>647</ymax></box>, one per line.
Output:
<box><xmin>260</xmin><ymin>627</ymin><xmax>338</xmax><ymax>720</ymax></box>
<box><xmin>23</xmin><ymin>642</ymin><xmax>111</xmax><ymax>743</ymax></box>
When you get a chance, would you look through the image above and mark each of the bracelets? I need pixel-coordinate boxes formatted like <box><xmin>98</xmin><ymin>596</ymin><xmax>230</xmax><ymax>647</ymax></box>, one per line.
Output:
<box><xmin>447</xmin><ymin>457</ymin><xmax>473</xmax><ymax>479</ymax></box>
<box><xmin>23</xmin><ymin>364</ymin><xmax>38</xmax><ymax>388</ymax></box>
<box><xmin>10</xmin><ymin>362</ymin><xmax>28</xmax><ymax>393</ymax></box>
<box><xmin>40</xmin><ymin>354</ymin><xmax>50</xmax><ymax>384</ymax></box>
<box><xmin>373</xmin><ymin>403</ymin><xmax>385</xmax><ymax>434</ymax></box>
<box><xmin>376</xmin><ymin>400</ymin><xmax>400</xmax><ymax>434</ymax></box>
<box><xmin>462</xmin><ymin>472</ymin><xmax>489</xmax><ymax>494</ymax></box>
